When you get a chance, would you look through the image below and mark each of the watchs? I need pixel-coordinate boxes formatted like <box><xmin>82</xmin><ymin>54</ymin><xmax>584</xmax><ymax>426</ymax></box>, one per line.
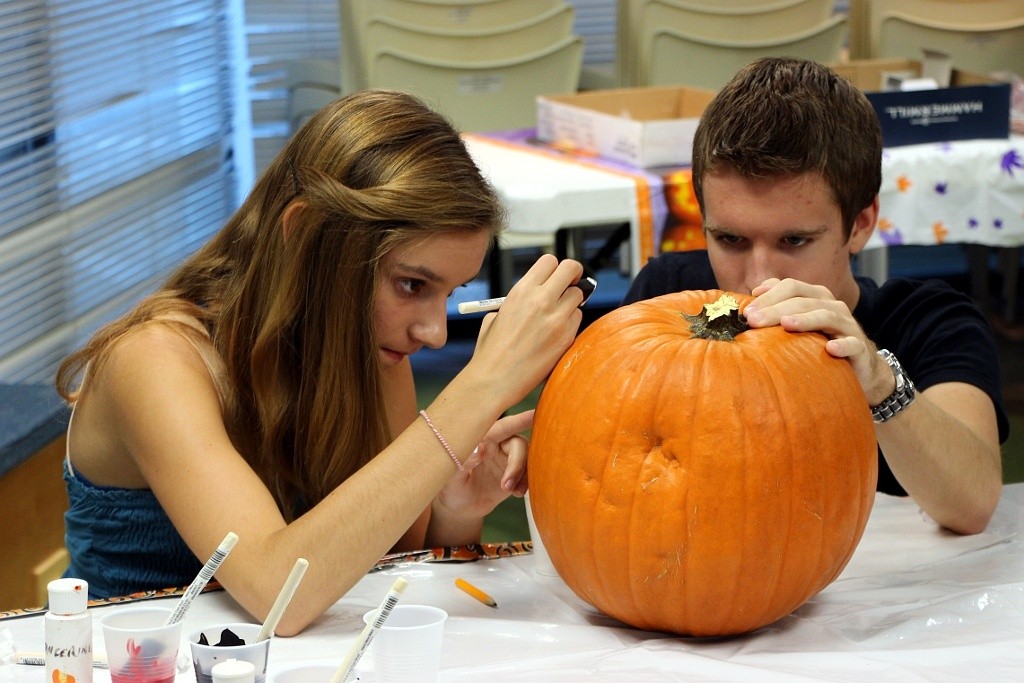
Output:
<box><xmin>868</xmin><ymin>348</ymin><xmax>916</xmax><ymax>425</ymax></box>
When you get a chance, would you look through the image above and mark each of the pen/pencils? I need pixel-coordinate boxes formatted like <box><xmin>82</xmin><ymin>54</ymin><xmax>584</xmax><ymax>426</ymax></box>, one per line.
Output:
<box><xmin>459</xmin><ymin>275</ymin><xmax>597</xmax><ymax>313</ymax></box>
<box><xmin>333</xmin><ymin>576</ymin><xmax>411</xmax><ymax>683</ymax></box>
<box><xmin>452</xmin><ymin>577</ymin><xmax>498</xmax><ymax>609</ymax></box>
<box><xmin>12</xmin><ymin>653</ymin><xmax>106</xmax><ymax>669</ymax></box>
<box><xmin>167</xmin><ymin>532</ymin><xmax>239</xmax><ymax>627</ymax></box>
<box><xmin>258</xmin><ymin>559</ymin><xmax>310</xmax><ymax>640</ymax></box>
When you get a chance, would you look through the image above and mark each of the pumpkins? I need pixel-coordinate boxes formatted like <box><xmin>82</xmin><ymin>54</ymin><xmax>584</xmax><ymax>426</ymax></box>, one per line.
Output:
<box><xmin>526</xmin><ymin>290</ymin><xmax>878</xmax><ymax>639</ymax></box>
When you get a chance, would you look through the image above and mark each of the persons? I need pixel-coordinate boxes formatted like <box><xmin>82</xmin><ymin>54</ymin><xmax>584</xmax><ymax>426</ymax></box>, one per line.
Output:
<box><xmin>624</xmin><ymin>58</ymin><xmax>1011</xmax><ymax>536</ymax></box>
<box><xmin>50</xmin><ymin>90</ymin><xmax>582</xmax><ymax>639</ymax></box>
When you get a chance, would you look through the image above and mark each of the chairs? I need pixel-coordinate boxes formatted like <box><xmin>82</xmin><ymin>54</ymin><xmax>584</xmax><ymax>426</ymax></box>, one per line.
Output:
<box><xmin>287</xmin><ymin>0</ymin><xmax>1024</xmax><ymax>134</ymax></box>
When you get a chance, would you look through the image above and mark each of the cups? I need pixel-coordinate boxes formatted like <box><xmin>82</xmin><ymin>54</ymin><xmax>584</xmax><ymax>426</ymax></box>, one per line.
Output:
<box><xmin>524</xmin><ymin>489</ymin><xmax>559</xmax><ymax>576</ymax></box>
<box><xmin>270</xmin><ymin>665</ymin><xmax>359</xmax><ymax>683</ymax></box>
<box><xmin>188</xmin><ymin>623</ymin><xmax>274</xmax><ymax>683</ymax></box>
<box><xmin>363</xmin><ymin>605</ymin><xmax>448</xmax><ymax>683</ymax></box>
<box><xmin>98</xmin><ymin>607</ymin><xmax>186</xmax><ymax>683</ymax></box>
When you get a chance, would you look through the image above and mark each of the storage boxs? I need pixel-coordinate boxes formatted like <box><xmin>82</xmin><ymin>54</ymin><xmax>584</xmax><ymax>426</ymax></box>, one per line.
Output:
<box><xmin>537</xmin><ymin>85</ymin><xmax>721</xmax><ymax>169</ymax></box>
<box><xmin>825</xmin><ymin>57</ymin><xmax>1012</xmax><ymax>147</ymax></box>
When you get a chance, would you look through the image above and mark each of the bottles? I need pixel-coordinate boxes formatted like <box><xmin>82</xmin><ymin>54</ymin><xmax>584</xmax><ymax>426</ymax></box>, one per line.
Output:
<box><xmin>211</xmin><ymin>658</ymin><xmax>255</xmax><ymax>683</ymax></box>
<box><xmin>44</xmin><ymin>578</ymin><xmax>93</xmax><ymax>683</ymax></box>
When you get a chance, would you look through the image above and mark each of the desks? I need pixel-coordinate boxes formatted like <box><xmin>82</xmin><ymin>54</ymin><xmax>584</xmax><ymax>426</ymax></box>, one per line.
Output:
<box><xmin>0</xmin><ymin>483</ymin><xmax>1024</xmax><ymax>682</ymax></box>
<box><xmin>457</xmin><ymin>128</ymin><xmax>1024</xmax><ymax>300</ymax></box>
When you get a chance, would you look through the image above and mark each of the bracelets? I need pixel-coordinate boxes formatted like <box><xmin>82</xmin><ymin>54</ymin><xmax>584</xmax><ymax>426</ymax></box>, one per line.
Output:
<box><xmin>419</xmin><ymin>410</ymin><xmax>466</xmax><ymax>474</ymax></box>
